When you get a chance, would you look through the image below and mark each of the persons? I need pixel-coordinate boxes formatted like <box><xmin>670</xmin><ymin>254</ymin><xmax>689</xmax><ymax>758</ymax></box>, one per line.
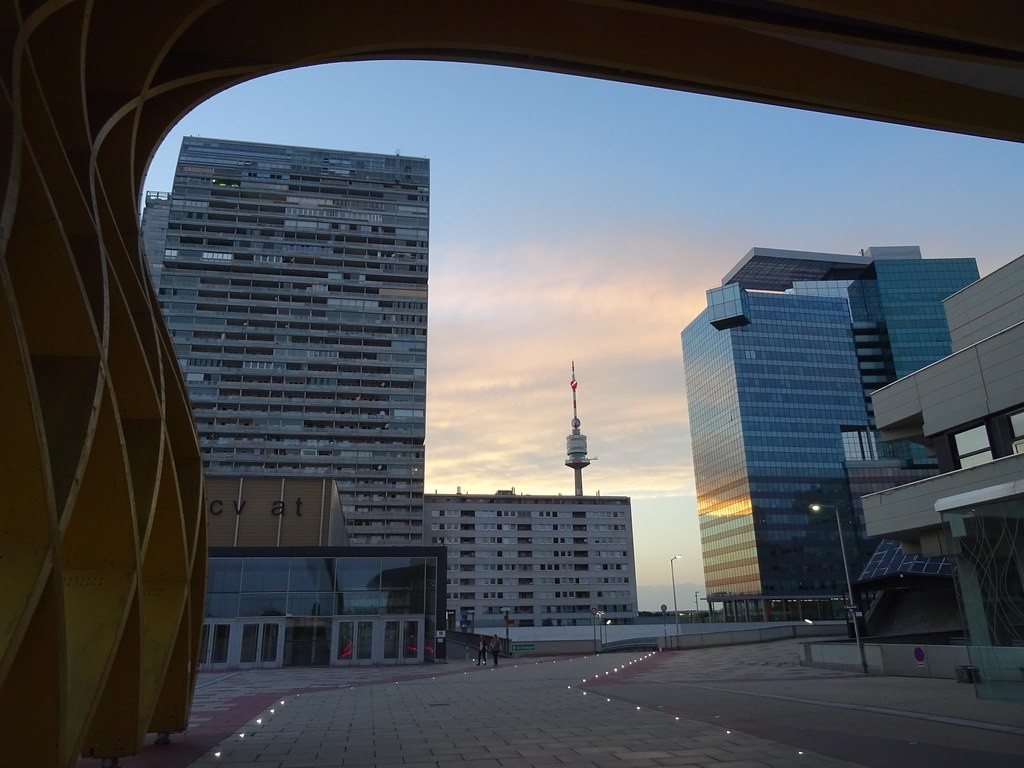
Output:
<box><xmin>476</xmin><ymin>634</ymin><xmax>488</xmax><ymax>666</ymax></box>
<box><xmin>490</xmin><ymin>633</ymin><xmax>503</xmax><ymax>667</ymax></box>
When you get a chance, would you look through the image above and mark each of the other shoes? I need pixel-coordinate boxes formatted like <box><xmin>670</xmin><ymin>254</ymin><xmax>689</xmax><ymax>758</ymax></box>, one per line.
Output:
<box><xmin>484</xmin><ymin>663</ymin><xmax>486</xmax><ymax>665</ymax></box>
<box><xmin>476</xmin><ymin>664</ymin><xmax>480</xmax><ymax>665</ymax></box>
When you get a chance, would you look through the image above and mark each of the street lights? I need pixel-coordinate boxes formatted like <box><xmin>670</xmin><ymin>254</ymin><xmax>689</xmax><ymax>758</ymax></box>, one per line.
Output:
<box><xmin>809</xmin><ymin>503</ymin><xmax>869</xmax><ymax>673</ymax></box>
<box><xmin>671</xmin><ymin>554</ymin><xmax>683</xmax><ymax>650</ymax></box>
<box><xmin>596</xmin><ymin>611</ymin><xmax>605</xmax><ymax>652</ymax></box>
<box><xmin>604</xmin><ymin>619</ymin><xmax>612</xmax><ymax>652</ymax></box>
